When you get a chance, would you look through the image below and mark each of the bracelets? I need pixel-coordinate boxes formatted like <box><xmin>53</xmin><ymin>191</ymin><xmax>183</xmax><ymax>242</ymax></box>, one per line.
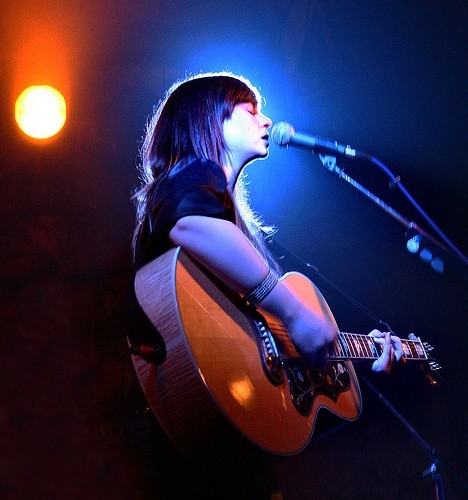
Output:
<box><xmin>241</xmin><ymin>266</ymin><xmax>279</xmax><ymax>303</ymax></box>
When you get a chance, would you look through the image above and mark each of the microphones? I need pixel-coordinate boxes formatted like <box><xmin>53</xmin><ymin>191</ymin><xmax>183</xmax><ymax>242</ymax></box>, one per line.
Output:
<box><xmin>272</xmin><ymin>121</ymin><xmax>373</xmax><ymax>162</ymax></box>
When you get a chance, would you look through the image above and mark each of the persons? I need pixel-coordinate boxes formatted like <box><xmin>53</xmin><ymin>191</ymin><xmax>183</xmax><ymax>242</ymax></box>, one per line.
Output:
<box><xmin>127</xmin><ymin>73</ymin><xmax>413</xmax><ymax>499</ymax></box>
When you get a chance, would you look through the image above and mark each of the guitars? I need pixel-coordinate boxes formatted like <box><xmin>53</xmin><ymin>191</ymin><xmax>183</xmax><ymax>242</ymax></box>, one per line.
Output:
<box><xmin>125</xmin><ymin>247</ymin><xmax>436</xmax><ymax>458</ymax></box>
